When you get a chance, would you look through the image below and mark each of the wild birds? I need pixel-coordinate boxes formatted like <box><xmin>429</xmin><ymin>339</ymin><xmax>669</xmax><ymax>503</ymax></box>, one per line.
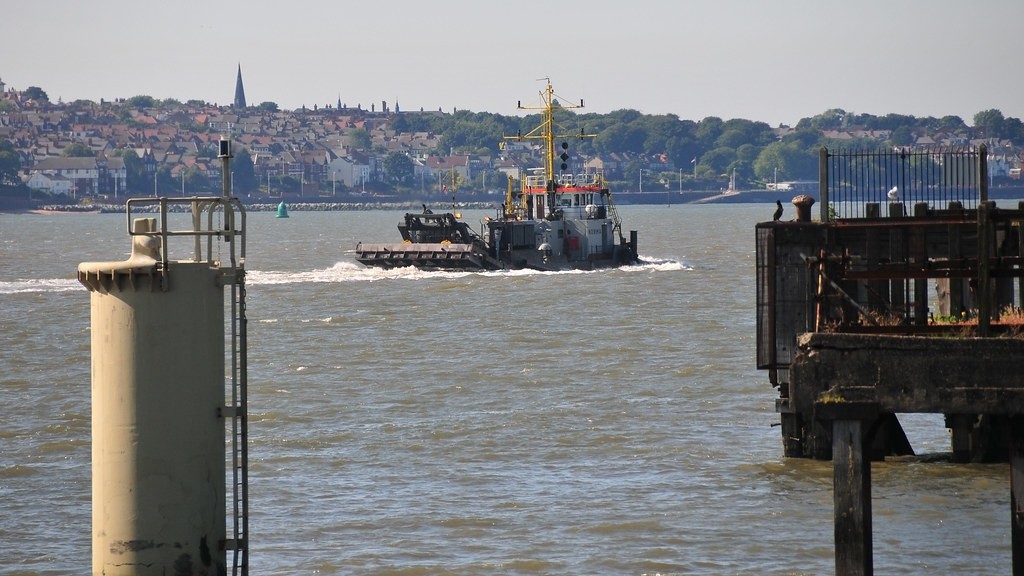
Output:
<box><xmin>888</xmin><ymin>185</ymin><xmax>899</xmax><ymax>206</ymax></box>
<box><xmin>772</xmin><ymin>200</ymin><xmax>783</xmax><ymax>222</ymax></box>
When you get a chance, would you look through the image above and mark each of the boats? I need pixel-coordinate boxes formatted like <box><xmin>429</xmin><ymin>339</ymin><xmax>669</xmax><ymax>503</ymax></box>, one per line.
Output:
<box><xmin>354</xmin><ymin>76</ymin><xmax>638</xmax><ymax>276</ymax></box>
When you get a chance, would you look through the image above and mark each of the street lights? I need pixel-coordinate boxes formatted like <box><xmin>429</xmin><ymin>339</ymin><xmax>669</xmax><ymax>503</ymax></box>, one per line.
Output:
<box><xmin>231</xmin><ymin>172</ymin><xmax>234</xmax><ymax>195</ymax></box>
<box><xmin>639</xmin><ymin>169</ymin><xmax>643</xmax><ymax>192</ymax></box>
<box><xmin>774</xmin><ymin>167</ymin><xmax>777</xmax><ymax>185</ymax></box>
<box><xmin>733</xmin><ymin>168</ymin><xmax>737</xmax><ymax>190</ymax></box>
<box><xmin>362</xmin><ymin>171</ymin><xmax>365</xmax><ymax>192</ymax></box>
<box><xmin>300</xmin><ymin>171</ymin><xmax>304</xmax><ymax>197</ymax></box>
<box><xmin>483</xmin><ymin>171</ymin><xmax>486</xmax><ymax>188</ymax></box>
<box><xmin>439</xmin><ymin>170</ymin><xmax>443</xmax><ymax>191</ymax></box>
<box><xmin>182</xmin><ymin>169</ymin><xmax>185</xmax><ymax>197</ymax></box>
<box><xmin>421</xmin><ymin>169</ymin><xmax>425</xmax><ymax>191</ymax></box>
<box><xmin>332</xmin><ymin>172</ymin><xmax>336</xmax><ymax>195</ymax></box>
<box><xmin>155</xmin><ymin>173</ymin><xmax>158</xmax><ymax>197</ymax></box>
<box><xmin>267</xmin><ymin>171</ymin><xmax>270</xmax><ymax>193</ymax></box>
<box><xmin>680</xmin><ymin>168</ymin><xmax>682</xmax><ymax>192</ymax></box>
